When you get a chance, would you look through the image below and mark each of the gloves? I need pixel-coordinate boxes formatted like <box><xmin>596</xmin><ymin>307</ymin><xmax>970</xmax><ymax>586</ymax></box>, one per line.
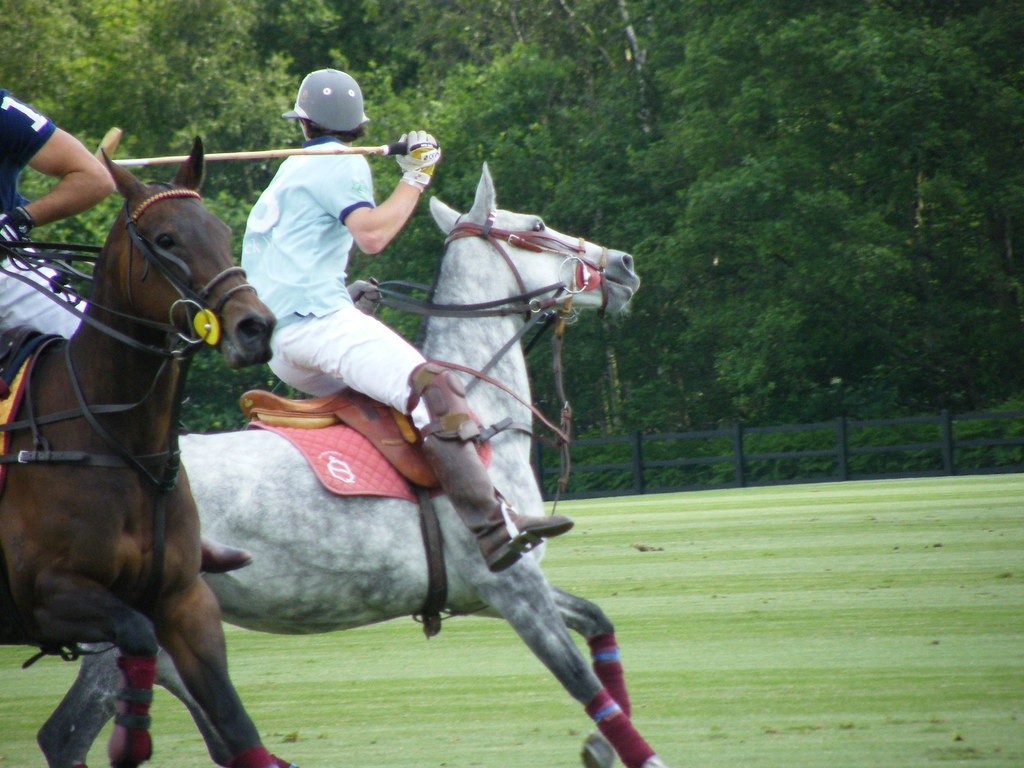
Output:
<box><xmin>395</xmin><ymin>130</ymin><xmax>441</xmax><ymax>186</ymax></box>
<box><xmin>0</xmin><ymin>206</ymin><xmax>37</xmax><ymax>261</ymax></box>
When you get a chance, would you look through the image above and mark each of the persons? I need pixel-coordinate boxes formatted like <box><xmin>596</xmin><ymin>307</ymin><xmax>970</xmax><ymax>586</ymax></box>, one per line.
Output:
<box><xmin>0</xmin><ymin>86</ymin><xmax>253</xmax><ymax>573</ymax></box>
<box><xmin>242</xmin><ymin>70</ymin><xmax>574</xmax><ymax>572</ymax></box>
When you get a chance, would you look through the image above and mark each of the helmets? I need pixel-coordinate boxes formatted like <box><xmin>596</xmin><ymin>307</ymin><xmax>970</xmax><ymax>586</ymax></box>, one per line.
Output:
<box><xmin>282</xmin><ymin>68</ymin><xmax>370</xmax><ymax>133</ymax></box>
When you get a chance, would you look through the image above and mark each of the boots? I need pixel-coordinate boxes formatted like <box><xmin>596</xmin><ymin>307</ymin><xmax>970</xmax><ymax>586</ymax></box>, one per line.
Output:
<box><xmin>420</xmin><ymin>433</ymin><xmax>575</xmax><ymax>574</ymax></box>
<box><xmin>199</xmin><ymin>531</ymin><xmax>254</xmax><ymax>574</ymax></box>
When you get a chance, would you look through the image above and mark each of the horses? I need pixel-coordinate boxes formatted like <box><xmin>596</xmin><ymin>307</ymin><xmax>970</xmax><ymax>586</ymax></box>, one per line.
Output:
<box><xmin>37</xmin><ymin>161</ymin><xmax>670</xmax><ymax>768</ymax></box>
<box><xmin>1</xmin><ymin>136</ymin><xmax>300</xmax><ymax>768</ymax></box>
